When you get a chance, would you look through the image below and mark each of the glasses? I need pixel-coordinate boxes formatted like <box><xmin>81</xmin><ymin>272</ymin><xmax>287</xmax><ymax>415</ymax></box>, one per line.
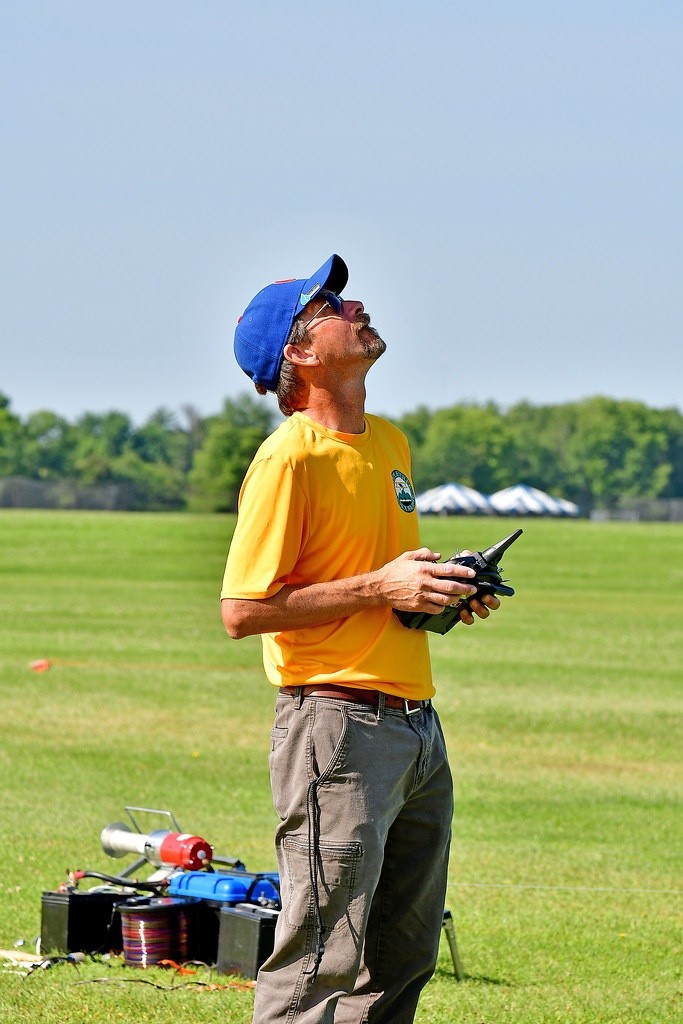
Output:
<box><xmin>303</xmin><ymin>292</ymin><xmax>345</xmax><ymax>332</ymax></box>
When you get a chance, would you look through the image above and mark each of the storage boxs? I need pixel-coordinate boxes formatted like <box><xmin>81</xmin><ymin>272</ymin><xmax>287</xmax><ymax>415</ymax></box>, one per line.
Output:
<box><xmin>40</xmin><ymin>892</ymin><xmax>142</xmax><ymax>959</ymax></box>
<box><xmin>218</xmin><ymin>905</ymin><xmax>278</xmax><ymax>979</ymax></box>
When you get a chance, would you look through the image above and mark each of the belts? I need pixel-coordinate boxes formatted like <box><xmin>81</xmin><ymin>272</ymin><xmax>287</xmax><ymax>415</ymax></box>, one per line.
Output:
<box><xmin>277</xmin><ymin>684</ymin><xmax>431</xmax><ymax>715</ymax></box>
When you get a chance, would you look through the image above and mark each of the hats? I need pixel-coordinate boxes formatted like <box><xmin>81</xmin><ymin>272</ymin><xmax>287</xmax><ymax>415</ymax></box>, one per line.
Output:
<box><xmin>233</xmin><ymin>253</ymin><xmax>349</xmax><ymax>393</ymax></box>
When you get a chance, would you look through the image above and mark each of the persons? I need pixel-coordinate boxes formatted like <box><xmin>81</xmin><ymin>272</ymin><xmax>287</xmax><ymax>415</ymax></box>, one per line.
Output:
<box><xmin>219</xmin><ymin>254</ymin><xmax>501</xmax><ymax>1023</ymax></box>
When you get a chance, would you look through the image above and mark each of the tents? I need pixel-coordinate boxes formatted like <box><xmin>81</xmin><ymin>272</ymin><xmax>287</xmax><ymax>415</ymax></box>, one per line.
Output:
<box><xmin>416</xmin><ymin>481</ymin><xmax>493</xmax><ymax>514</ymax></box>
<box><xmin>489</xmin><ymin>483</ymin><xmax>579</xmax><ymax>515</ymax></box>
